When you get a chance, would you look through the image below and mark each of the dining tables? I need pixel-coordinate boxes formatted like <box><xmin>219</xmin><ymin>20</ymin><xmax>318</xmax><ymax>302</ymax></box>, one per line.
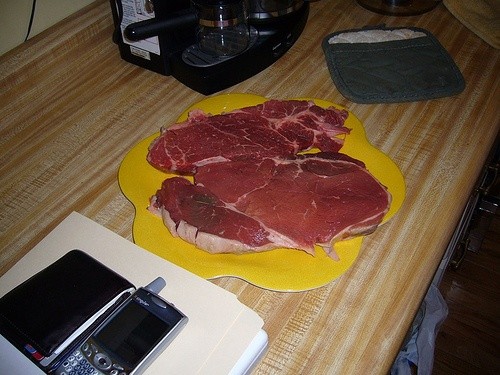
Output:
<box><xmin>0</xmin><ymin>0</ymin><xmax>500</xmax><ymax>374</ymax></box>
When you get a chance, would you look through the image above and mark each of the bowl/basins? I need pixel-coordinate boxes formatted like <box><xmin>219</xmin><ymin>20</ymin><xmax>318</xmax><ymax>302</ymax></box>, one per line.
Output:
<box><xmin>356</xmin><ymin>0</ymin><xmax>442</xmax><ymax>16</ymax></box>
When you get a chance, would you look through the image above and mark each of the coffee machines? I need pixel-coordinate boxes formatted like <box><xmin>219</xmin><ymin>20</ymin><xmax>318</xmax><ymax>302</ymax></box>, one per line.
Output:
<box><xmin>109</xmin><ymin>0</ymin><xmax>310</xmax><ymax>97</ymax></box>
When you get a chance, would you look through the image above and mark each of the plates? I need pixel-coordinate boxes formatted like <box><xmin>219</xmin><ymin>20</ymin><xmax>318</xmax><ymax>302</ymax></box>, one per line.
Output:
<box><xmin>118</xmin><ymin>93</ymin><xmax>407</xmax><ymax>292</ymax></box>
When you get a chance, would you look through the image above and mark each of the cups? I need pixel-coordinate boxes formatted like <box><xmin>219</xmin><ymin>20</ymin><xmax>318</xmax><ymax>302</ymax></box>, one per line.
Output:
<box><xmin>192</xmin><ymin>0</ymin><xmax>250</xmax><ymax>56</ymax></box>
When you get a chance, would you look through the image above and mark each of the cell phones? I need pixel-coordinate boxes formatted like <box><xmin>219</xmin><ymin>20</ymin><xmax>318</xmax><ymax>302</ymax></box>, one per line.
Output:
<box><xmin>45</xmin><ymin>277</ymin><xmax>189</xmax><ymax>375</ymax></box>
